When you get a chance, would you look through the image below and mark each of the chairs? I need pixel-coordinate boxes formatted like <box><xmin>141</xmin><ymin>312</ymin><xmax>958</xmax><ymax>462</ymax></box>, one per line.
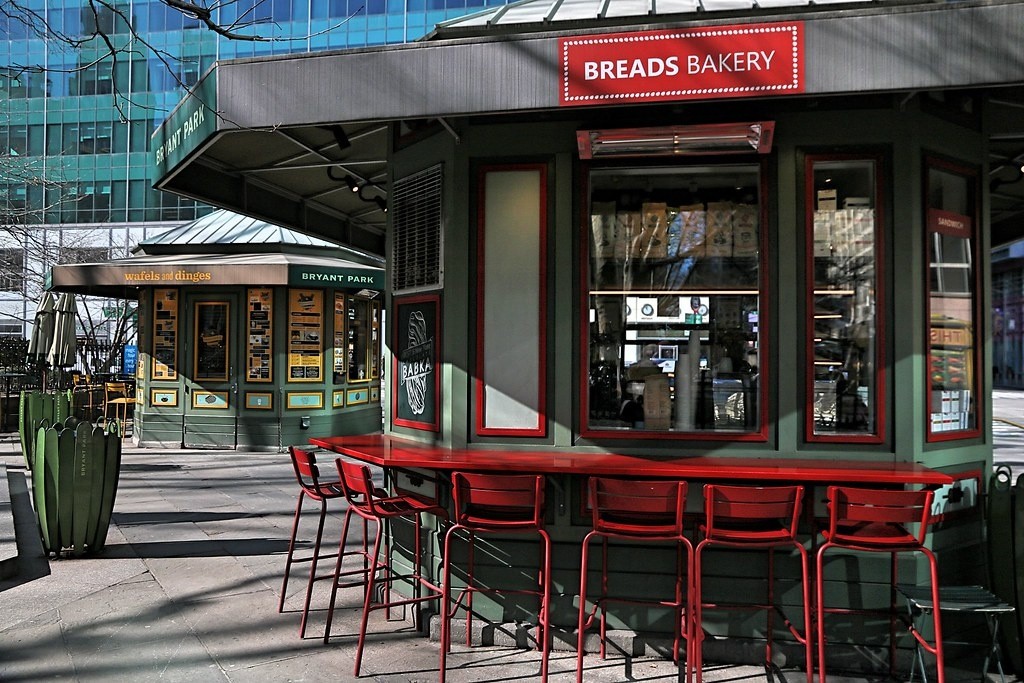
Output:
<box><xmin>104</xmin><ymin>382</ymin><xmax>136</xmax><ymax>443</ymax></box>
<box><xmin>73</xmin><ymin>375</ymin><xmax>106</xmax><ymax>421</ymax></box>
<box><xmin>278</xmin><ymin>447</ymin><xmax>946</xmax><ymax>683</ymax></box>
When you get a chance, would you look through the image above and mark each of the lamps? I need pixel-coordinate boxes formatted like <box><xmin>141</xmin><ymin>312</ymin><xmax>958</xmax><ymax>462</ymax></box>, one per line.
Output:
<box><xmin>327</xmin><ymin>159</ymin><xmax>359</xmax><ymax>193</ymax></box>
<box><xmin>357</xmin><ymin>181</ymin><xmax>388</xmax><ymax>213</ymax></box>
<box><xmin>988</xmin><ymin>160</ymin><xmax>1023</xmax><ymax>193</ymax></box>
<box><xmin>314</xmin><ymin>126</ymin><xmax>350</xmax><ymax>150</ymax></box>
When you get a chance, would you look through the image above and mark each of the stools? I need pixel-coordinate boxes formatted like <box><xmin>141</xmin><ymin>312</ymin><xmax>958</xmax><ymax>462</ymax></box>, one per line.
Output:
<box><xmin>893</xmin><ymin>585</ymin><xmax>1015</xmax><ymax>683</ymax></box>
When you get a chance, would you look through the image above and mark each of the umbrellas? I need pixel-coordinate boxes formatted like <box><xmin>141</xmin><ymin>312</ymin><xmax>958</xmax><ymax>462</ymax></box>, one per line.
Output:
<box><xmin>25</xmin><ymin>287</ymin><xmax>56</xmax><ymax>395</ymax></box>
<box><xmin>46</xmin><ymin>292</ymin><xmax>79</xmax><ymax>390</ymax></box>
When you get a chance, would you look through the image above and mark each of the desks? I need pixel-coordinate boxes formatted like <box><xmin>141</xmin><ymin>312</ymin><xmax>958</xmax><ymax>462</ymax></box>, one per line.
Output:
<box><xmin>95</xmin><ymin>372</ymin><xmax>135</xmax><ymax>418</ymax></box>
<box><xmin>0</xmin><ymin>373</ymin><xmax>27</xmax><ymax>431</ymax></box>
<box><xmin>309</xmin><ymin>436</ymin><xmax>951</xmax><ymax>485</ymax></box>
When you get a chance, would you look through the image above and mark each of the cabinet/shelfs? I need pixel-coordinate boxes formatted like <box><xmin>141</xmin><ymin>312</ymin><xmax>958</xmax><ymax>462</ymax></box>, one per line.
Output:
<box><xmin>588</xmin><ymin>253</ymin><xmax>874</xmax><ymax>381</ymax></box>
